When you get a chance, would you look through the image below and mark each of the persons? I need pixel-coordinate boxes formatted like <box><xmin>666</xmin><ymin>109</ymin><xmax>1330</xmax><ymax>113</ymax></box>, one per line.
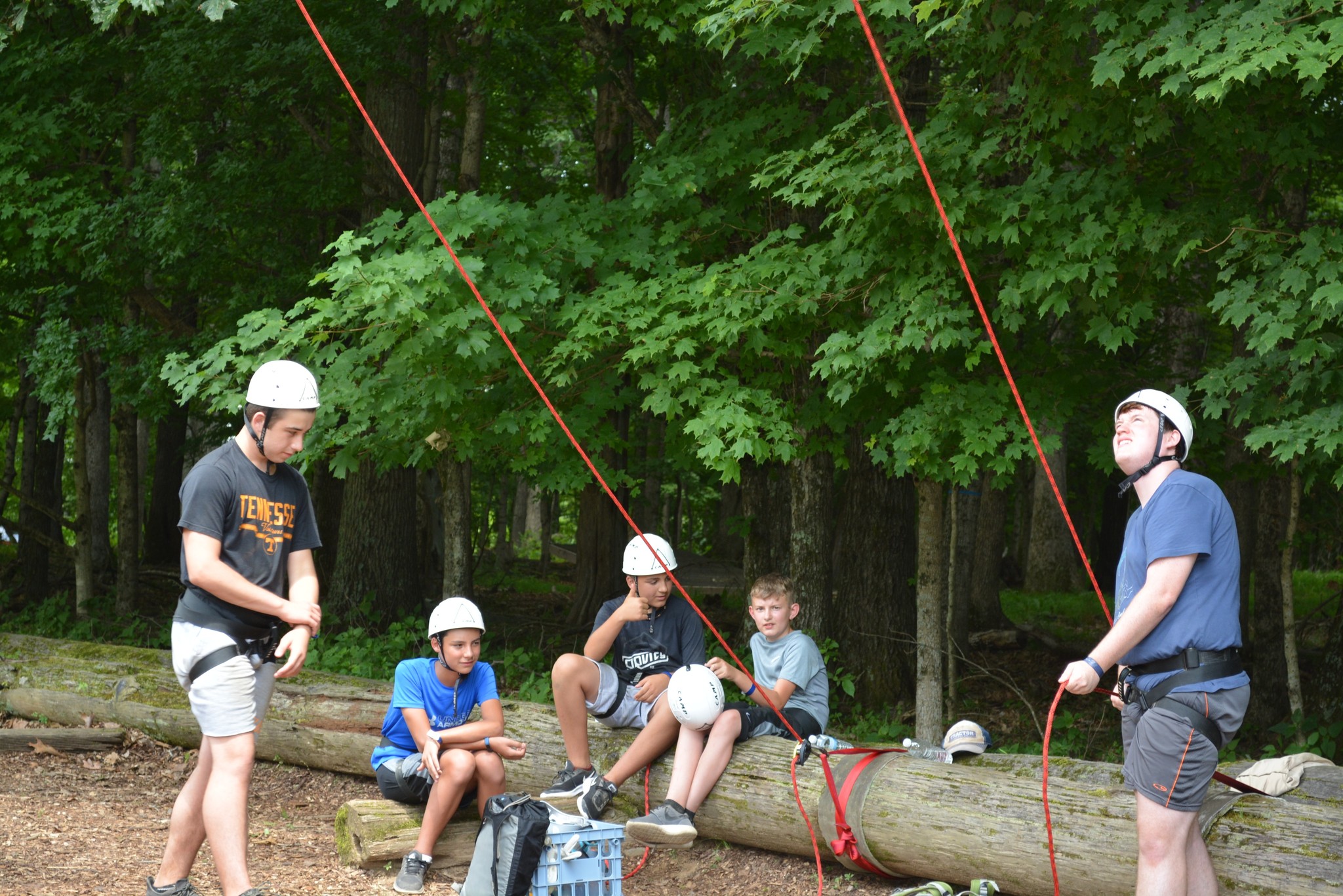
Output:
<box><xmin>371</xmin><ymin>597</ymin><xmax>529</xmax><ymax>894</ymax></box>
<box><xmin>540</xmin><ymin>533</ymin><xmax>706</xmax><ymax>822</ymax></box>
<box><xmin>625</xmin><ymin>575</ymin><xmax>831</xmax><ymax>852</ymax></box>
<box><xmin>1057</xmin><ymin>388</ymin><xmax>1252</xmax><ymax>896</ymax></box>
<box><xmin>146</xmin><ymin>359</ymin><xmax>325</xmax><ymax>896</ymax></box>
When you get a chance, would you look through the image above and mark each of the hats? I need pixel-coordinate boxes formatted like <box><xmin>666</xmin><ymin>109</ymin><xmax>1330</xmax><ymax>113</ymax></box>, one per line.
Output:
<box><xmin>941</xmin><ymin>720</ymin><xmax>993</xmax><ymax>754</ymax></box>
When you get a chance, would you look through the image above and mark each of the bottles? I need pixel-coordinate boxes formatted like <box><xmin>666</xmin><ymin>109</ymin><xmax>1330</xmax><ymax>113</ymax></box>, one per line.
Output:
<box><xmin>903</xmin><ymin>738</ymin><xmax>953</xmax><ymax>765</ymax></box>
<box><xmin>808</xmin><ymin>734</ymin><xmax>854</xmax><ymax>752</ymax></box>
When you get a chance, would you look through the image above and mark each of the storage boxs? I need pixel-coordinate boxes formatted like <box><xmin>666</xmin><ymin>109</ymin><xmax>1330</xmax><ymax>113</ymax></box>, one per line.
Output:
<box><xmin>525</xmin><ymin>819</ymin><xmax>626</xmax><ymax>896</ymax></box>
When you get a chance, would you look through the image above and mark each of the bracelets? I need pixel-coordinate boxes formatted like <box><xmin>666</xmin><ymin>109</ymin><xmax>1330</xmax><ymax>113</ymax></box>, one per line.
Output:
<box><xmin>1083</xmin><ymin>656</ymin><xmax>1105</xmax><ymax>679</ymax></box>
<box><xmin>743</xmin><ymin>682</ymin><xmax>756</xmax><ymax>696</ymax></box>
<box><xmin>661</xmin><ymin>671</ymin><xmax>672</xmax><ymax>679</ymax></box>
<box><xmin>484</xmin><ymin>737</ymin><xmax>492</xmax><ymax>753</ymax></box>
<box><xmin>426</xmin><ymin>729</ymin><xmax>442</xmax><ymax>745</ymax></box>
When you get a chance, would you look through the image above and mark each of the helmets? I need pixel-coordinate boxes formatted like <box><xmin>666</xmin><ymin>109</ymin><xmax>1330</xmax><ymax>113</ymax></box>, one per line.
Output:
<box><xmin>1115</xmin><ymin>389</ymin><xmax>1193</xmax><ymax>462</ymax></box>
<box><xmin>427</xmin><ymin>597</ymin><xmax>486</xmax><ymax>640</ymax></box>
<box><xmin>246</xmin><ymin>360</ymin><xmax>320</xmax><ymax>409</ymax></box>
<box><xmin>668</xmin><ymin>664</ymin><xmax>725</xmax><ymax>731</ymax></box>
<box><xmin>622</xmin><ymin>534</ymin><xmax>677</xmax><ymax>576</ymax></box>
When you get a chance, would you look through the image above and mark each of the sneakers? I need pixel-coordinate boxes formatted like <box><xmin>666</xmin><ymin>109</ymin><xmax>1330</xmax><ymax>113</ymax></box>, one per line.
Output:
<box><xmin>145</xmin><ymin>876</ymin><xmax>198</xmax><ymax>896</ymax></box>
<box><xmin>577</xmin><ymin>775</ymin><xmax>618</xmax><ymax>820</ymax></box>
<box><xmin>626</xmin><ymin>804</ymin><xmax>698</xmax><ymax>848</ymax></box>
<box><xmin>539</xmin><ymin>761</ymin><xmax>597</xmax><ymax>797</ymax></box>
<box><xmin>237</xmin><ymin>888</ymin><xmax>265</xmax><ymax>896</ymax></box>
<box><xmin>393</xmin><ymin>850</ymin><xmax>433</xmax><ymax>894</ymax></box>
